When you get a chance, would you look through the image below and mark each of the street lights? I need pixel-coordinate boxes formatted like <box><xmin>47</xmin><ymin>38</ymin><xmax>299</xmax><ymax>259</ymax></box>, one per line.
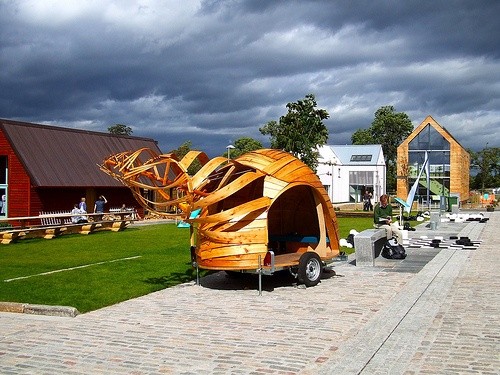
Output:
<box><xmin>225</xmin><ymin>145</ymin><xmax>236</xmax><ymax>165</ymax></box>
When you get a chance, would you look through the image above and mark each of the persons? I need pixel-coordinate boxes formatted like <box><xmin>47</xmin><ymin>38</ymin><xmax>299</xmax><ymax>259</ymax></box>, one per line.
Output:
<box><xmin>93</xmin><ymin>195</ymin><xmax>107</xmax><ymax>220</ymax></box>
<box><xmin>120</xmin><ymin>204</ymin><xmax>127</xmax><ymax>212</ymax></box>
<box><xmin>363</xmin><ymin>191</ymin><xmax>373</xmax><ymax>211</ymax></box>
<box><xmin>79</xmin><ymin>198</ymin><xmax>86</xmax><ymax>211</ymax></box>
<box><xmin>71</xmin><ymin>204</ymin><xmax>89</xmax><ymax>223</ymax></box>
<box><xmin>373</xmin><ymin>194</ymin><xmax>403</xmax><ymax>245</ymax></box>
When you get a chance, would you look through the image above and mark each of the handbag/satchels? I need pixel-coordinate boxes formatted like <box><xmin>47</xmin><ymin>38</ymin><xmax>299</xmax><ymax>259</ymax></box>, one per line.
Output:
<box><xmin>80</xmin><ymin>203</ymin><xmax>86</xmax><ymax>212</ymax></box>
<box><xmin>370</xmin><ymin>199</ymin><xmax>375</xmax><ymax>205</ymax></box>
<box><xmin>381</xmin><ymin>238</ymin><xmax>407</xmax><ymax>260</ymax></box>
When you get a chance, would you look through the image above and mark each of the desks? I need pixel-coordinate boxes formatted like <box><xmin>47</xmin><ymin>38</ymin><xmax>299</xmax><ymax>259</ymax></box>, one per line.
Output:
<box><xmin>0</xmin><ymin>211</ymin><xmax>131</xmax><ymax>230</ymax></box>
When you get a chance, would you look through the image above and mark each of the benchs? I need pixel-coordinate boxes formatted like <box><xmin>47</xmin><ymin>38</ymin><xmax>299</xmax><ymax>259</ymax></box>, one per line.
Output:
<box><xmin>431</xmin><ymin>212</ymin><xmax>441</xmax><ymax>225</ymax></box>
<box><xmin>353</xmin><ymin>227</ymin><xmax>386</xmax><ymax>266</ymax></box>
<box><xmin>0</xmin><ymin>219</ymin><xmax>139</xmax><ymax>245</ymax></box>
